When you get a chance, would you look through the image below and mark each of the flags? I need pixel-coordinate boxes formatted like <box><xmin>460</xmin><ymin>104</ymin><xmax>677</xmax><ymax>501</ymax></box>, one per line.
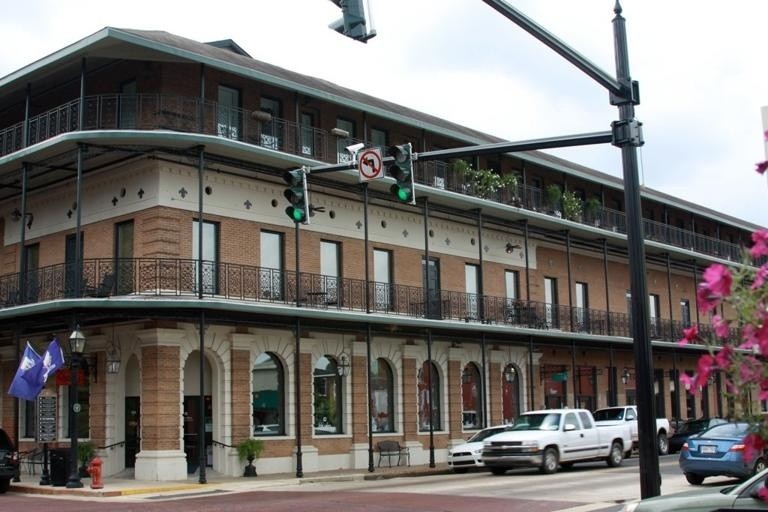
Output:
<box><xmin>6</xmin><ymin>343</ymin><xmax>46</xmax><ymax>401</ymax></box>
<box><xmin>18</xmin><ymin>337</ymin><xmax>66</xmax><ymax>394</ymax></box>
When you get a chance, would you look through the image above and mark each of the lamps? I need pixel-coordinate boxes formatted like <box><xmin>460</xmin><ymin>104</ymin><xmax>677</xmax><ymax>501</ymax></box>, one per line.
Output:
<box><xmin>504</xmin><ymin>337</ymin><xmax>516</xmax><ymax>384</ymax></box>
<box><xmin>104</xmin><ymin>324</ymin><xmax>123</xmax><ymax>375</ymax></box>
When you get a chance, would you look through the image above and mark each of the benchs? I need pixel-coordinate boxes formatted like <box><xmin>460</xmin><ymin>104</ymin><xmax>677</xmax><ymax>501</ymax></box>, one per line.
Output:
<box><xmin>18</xmin><ymin>442</ymin><xmax>51</xmax><ymax>475</ymax></box>
<box><xmin>376</xmin><ymin>440</ymin><xmax>411</xmax><ymax>469</ymax></box>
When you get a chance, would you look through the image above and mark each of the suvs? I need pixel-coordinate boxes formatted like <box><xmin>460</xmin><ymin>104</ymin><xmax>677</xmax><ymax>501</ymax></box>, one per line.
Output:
<box><xmin>0</xmin><ymin>429</ymin><xmax>19</xmax><ymax>492</ymax></box>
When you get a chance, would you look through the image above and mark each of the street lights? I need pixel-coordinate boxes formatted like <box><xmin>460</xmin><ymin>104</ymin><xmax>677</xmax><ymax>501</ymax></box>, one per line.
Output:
<box><xmin>63</xmin><ymin>323</ymin><xmax>89</xmax><ymax>489</ymax></box>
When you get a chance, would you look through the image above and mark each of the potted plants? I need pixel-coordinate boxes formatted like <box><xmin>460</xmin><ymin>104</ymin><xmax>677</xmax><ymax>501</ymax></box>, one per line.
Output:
<box><xmin>233</xmin><ymin>436</ymin><xmax>264</xmax><ymax>478</ymax></box>
<box><xmin>77</xmin><ymin>440</ymin><xmax>97</xmax><ymax>478</ymax></box>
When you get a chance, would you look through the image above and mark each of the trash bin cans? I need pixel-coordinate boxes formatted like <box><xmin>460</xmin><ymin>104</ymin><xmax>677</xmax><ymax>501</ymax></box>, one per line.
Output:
<box><xmin>50</xmin><ymin>447</ymin><xmax>74</xmax><ymax>486</ymax></box>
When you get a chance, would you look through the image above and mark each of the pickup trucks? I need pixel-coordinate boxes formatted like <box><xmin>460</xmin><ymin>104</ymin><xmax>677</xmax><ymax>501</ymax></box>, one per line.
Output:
<box><xmin>481</xmin><ymin>407</ymin><xmax>632</xmax><ymax>474</ymax></box>
<box><xmin>589</xmin><ymin>405</ymin><xmax>672</xmax><ymax>455</ymax></box>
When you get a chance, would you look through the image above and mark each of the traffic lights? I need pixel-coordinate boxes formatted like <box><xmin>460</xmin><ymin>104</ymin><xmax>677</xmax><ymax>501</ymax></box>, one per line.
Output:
<box><xmin>388</xmin><ymin>139</ymin><xmax>416</xmax><ymax>208</ymax></box>
<box><xmin>330</xmin><ymin>0</ymin><xmax>367</xmax><ymax>43</ymax></box>
<box><xmin>283</xmin><ymin>163</ymin><xmax>311</xmax><ymax>225</ymax></box>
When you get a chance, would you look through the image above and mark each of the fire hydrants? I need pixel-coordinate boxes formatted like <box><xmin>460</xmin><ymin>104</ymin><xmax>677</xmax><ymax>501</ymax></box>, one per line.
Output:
<box><xmin>86</xmin><ymin>456</ymin><xmax>105</xmax><ymax>490</ymax></box>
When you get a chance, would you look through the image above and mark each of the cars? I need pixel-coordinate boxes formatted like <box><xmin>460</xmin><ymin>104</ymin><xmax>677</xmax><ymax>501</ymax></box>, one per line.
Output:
<box><xmin>666</xmin><ymin>418</ymin><xmax>728</xmax><ymax>451</ymax></box>
<box><xmin>616</xmin><ymin>467</ymin><xmax>768</xmax><ymax>511</ymax></box>
<box><xmin>249</xmin><ymin>420</ymin><xmax>336</xmax><ymax>436</ymax></box>
<box><xmin>446</xmin><ymin>423</ymin><xmax>512</xmax><ymax>470</ymax></box>
<box><xmin>681</xmin><ymin>422</ymin><xmax>767</xmax><ymax>486</ymax></box>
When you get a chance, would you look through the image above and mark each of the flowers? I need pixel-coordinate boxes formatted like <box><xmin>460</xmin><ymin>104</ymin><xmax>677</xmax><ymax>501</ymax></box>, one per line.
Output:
<box><xmin>676</xmin><ymin>229</ymin><xmax>767</xmax><ymax>500</ymax></box>
